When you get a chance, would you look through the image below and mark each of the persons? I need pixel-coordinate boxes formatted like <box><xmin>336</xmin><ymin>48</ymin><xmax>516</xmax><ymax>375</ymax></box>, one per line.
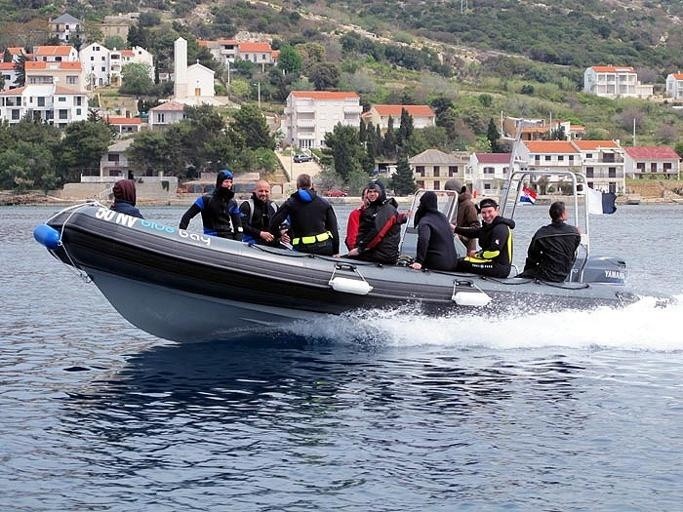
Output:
<box><xmin>109</xmin><ymin>179</ymin><xmax>144</xmax><ymax>219</ymax></box>
<box><xmin>341</xmin><ymin>182</ymin><xmax>401</xmax><ymax>266</ymax></box>
<box><xmin>179</xmin><ymin>170</ymin><xmax>244</xmax><ymax>242</ymax></box>
<box><xmin>445</xmin><ymin>178</ymin><xmax>481</xmax><ymax>258</ymax></box>
<box><xmin>238</xmin><ymin>179</ymin><xmax>293</xmax><ymax>250</ymax></box>
<box><xmin>449</xmin><ymin>198</ymin><xmax>515</xmax><ymax>278</ymax></box>
<box><xmin>270</xmin><ymin>173</ymin><xmax>340</xmax><ymax>259</ymax></box>
<box><xmin>513</xmin><ymin>201</ymin><xmax>581</xmax><ymax>283</ymax></box>
<box><xmin>409</xmin><ymin>191</ymin><xmax>457</xmax><ymax>271</ymax></box>
<box><xmin>344</xmin><ymin>186</ymin><xmax>415</xmax><ymax>252</ymax></box>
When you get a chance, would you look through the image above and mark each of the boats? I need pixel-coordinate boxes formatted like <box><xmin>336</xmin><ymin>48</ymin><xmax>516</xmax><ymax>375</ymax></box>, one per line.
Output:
<box><xmin>32</xmin><ymin>203</ymin><xmax>643</xmax><ymax>345</ymax></box>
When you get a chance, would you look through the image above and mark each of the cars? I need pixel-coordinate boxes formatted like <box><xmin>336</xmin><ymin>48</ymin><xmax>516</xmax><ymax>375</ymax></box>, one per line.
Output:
<box><xmin>293</xmin><ymin>154</ymin><xmax>311</xmax><ymax>163</ymax></box>
<box><xmin>323</xmin><ymin>189</ymin><xmax>347</xmax><ymax>197</ymax></box>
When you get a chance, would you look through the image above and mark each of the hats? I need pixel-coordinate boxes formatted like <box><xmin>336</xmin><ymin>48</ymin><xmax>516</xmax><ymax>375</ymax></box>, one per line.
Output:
<box><xmin>444</xmin><ymin>178</ymin><xmax>461</xmax><ymax>193</ymax></box>
<box><xmin>419</xmin><ymin>191</ymin><xmax>437</xmax><ymax>210</ymax></box>
<box><xmin>479</xmin><ymin>198</ymin><xmax>497</xmax><ymax>209</ymax></box>
